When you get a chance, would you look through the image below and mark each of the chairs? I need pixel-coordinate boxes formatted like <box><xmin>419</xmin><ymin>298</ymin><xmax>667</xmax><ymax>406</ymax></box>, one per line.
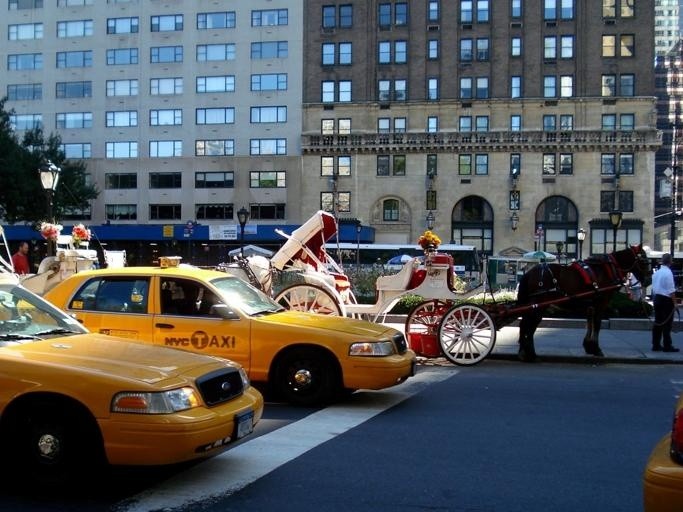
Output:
<box><xmin>374</xmin><ymin>257</ymin><xmax>421</xmax><ymax>291</ymax></box>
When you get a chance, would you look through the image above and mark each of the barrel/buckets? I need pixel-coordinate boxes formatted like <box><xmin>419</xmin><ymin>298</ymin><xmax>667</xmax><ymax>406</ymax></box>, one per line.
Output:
<box><xmin>406</xmin><ymin>332</ymin><xmax>423</xmax><ymax>354</ymax></box>
<box><xmin>421</xmin><ymin>333</ymin><xmax>440</xmax><ymax>357</ymax></box>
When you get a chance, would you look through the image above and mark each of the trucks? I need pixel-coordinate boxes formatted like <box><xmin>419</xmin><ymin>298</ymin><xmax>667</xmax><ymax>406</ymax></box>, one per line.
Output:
<box><xmin>53</xmin><ymin>233</ymin><xmax>89</xmax><ymax>258</ymax></box>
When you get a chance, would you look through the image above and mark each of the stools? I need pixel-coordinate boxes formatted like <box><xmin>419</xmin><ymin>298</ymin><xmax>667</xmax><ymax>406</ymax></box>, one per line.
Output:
<box><xmin>325</xmin><ymin>272</ymin><xmax>352</xmax><ymax>290</ymax></box>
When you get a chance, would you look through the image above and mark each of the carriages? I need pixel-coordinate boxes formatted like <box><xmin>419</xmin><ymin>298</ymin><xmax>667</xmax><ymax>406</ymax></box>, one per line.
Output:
<box><xmin>265</xmin><ymin>212</ymin><xmax>655</xmax><ymax>369</ymax></box>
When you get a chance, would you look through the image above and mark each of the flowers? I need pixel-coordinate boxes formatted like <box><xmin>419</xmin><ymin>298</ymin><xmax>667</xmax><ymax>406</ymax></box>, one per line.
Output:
<box><xmin>71</xmin><ymin>223</ymin><xmax>92</xmax><ymax>244</ymax></box>
<box><xmin>417</xmin><ymin>230</ymin><xmax>440</xmax><ymax>247</ymax></box>
<box><xmin>38</xmin><ymin>220</ymin><xmax>63</xmax><ymax>241</ymax></box>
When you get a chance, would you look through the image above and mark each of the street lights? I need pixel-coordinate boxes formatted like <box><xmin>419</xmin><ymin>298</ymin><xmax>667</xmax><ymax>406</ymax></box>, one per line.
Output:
<box><xmin>37</xmin><ymin>160</ymin><xmax>61</xmax><ymax>261</ymax></box>
<box><xmin>607</xmin><ymin>209</ymin><xmax>624</xmax><ymax>253</ymax></box>
<box><xmin>184</xmin><ymin>219</ymin><xmax>196</xmax><ymax>261</ymax></box>
<box><xmin>575</xmin><ymin>228</ymin><xmax>587</xmax><ymax>262</ymax></box>
<box><xmin>236</xmin><ymin>204</ymin><xmax>251</xmax><ymax>260</ymax></box>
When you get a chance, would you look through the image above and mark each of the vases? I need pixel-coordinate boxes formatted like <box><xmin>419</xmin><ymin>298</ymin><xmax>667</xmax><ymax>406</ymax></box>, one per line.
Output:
<box><xmin>423</xmin><ymin>243</ymin><xmax>438</xmax><ymax>257</ymax></box>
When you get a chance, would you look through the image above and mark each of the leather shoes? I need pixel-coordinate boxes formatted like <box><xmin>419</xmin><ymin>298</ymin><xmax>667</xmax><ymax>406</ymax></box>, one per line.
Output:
<box><xmin>651</xmin><ymin>346</ymin><xmax>663</xmax><ymax>352</ymax></box>
<box><xmin>663</xmin><ymin>347</ymin><xmax>680</xmax><ymax>353</ymax></box>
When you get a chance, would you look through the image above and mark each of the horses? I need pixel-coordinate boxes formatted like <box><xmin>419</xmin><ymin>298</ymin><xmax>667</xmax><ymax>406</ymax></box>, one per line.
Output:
<box><xmin>517</xmin><ymin>242</ymin><xmax>653</xmax><ymax>357</ymax></box>
<box><xmin>224</xmin><ymin>255</ymin><xmax>273</xmax><ymax>296</ymax></box>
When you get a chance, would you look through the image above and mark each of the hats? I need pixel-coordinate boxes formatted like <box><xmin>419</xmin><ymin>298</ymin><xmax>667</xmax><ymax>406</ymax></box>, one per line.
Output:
<box><xmin>658</xmin><ymin>253</ymin><xmax>675</xmax><ymax>261</ymax></box>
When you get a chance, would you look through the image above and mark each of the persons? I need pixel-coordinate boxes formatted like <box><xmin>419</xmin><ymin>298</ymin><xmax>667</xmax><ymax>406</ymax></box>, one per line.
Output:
<box><xmin>8</xmin><ymin>241</ymin><xmax>29</xmax><ymax>274</ymax></box>
<box><xmin>628</xmin><ymin>271</ymin><xmax>642</xmax><ymax>302</ymax></box>
<box><xmin>651</xmin><ymin>253</ymin><xmax>679</xmax><ymax>352</ymax></box>
<box><xmin>622</xmin><ymin>272</ymin><xmax>632</xmax><ymax>299</ymax></box>
